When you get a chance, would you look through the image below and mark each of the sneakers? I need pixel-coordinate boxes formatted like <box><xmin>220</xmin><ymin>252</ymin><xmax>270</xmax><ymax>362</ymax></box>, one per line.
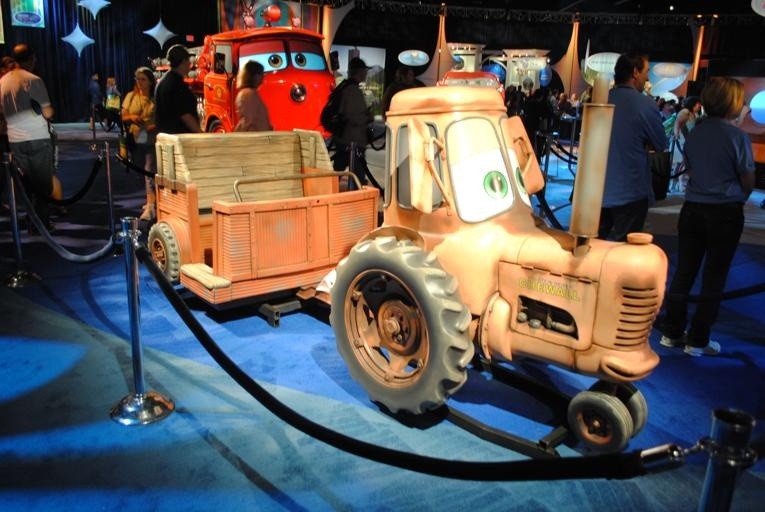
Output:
<box><xmin>683</xmin><ymin>340</ymin><xmax>721</xmax><ymax>357</ymax></box>
<box><xmin>139</xmin><ymin>205</ymin><xmax>157</xmax><ymax>221</ymax></box>
<box><xmin>27</xmin><ymin>224</ymin><xmax>67</xmax><ymax>236</ymax></box>
<box><xmin>660</xmin><ymin>331</ymin><xmax>689</xmax><ymax>349</ymax></box>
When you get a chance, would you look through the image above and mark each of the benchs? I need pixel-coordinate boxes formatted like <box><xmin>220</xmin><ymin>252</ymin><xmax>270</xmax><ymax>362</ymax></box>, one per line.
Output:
<box><xmin>157</xmin><ymin>129</ymin><xmax>306</xmax><ymax>248</ymax></box>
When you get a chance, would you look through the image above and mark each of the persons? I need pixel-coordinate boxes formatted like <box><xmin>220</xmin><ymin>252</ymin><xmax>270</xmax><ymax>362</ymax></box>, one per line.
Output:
<box><xmin>598</xmin><ymin>48</ymin><xmax>669</xmax><ymax>242</ymax></box>
<box><xmin>228</xmin><ymin>59</ymin><xmax>274</xmax><ymax>133</ymax></box>
<box><xmin>118</xmin><ymin>67</ymin><xmax>161</xmax><ymax>223</ymax></box>
<box><xmin>0</xmin><ymin>43</ymin><xmax>68</xmax><ymax>239</ymax></box>
<box><xmin>380</xmin><ymin>61</ymin><xmax>426</xmax><ymax>118</ymax></box>
<box><xmin>87</xmin><ymin>71</ymin><xmax>102</xmax><ymax>133</ymax></box>
<box><xmin>334</xmin><ymin>56</ymin><xmax>376</xmax><ymax>191</ymax></box>
<box><xmin>504</xmin><ymin>81</ymin><xmax>705</xmax><ymax>156</ymax></box>
<box><xmin>153</xmin><ymin>43</ymin><xmax>204</xmax><ymax>132</ymax></box>
<box><xmin>103</xmin><ymin>77</ymin><xmax>120</xmax><ymax>132</ymax></box>
<box><xmin>652</xmin><ymin>73</ymin><xmax>758</xmax><ymax>357</ymax></box>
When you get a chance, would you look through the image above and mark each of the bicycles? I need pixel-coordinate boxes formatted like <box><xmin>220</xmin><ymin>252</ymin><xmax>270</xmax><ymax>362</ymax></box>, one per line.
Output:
<box><xmin>99</xmin><ymin>102</ymin><xmax>122</xmax><ymax>132</ymax></box>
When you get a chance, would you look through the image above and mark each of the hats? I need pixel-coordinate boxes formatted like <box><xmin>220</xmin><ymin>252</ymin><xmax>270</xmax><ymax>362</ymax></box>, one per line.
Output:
<box><xmin>348</xmin><ymin>57</ymin><xmax>373</xmax><ymax>71</ymax></box>
<box><xmin>166</xmin><ymin>44</ymin><xmax>196</xmax><ymax>60</ymax></box>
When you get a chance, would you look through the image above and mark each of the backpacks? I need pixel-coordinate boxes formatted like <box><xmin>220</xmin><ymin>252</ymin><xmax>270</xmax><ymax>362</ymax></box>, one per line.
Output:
<box><xmin>321</xmin><ymin>78</ymin><xmax>356</xmax><ymax>134</ymax></box>
